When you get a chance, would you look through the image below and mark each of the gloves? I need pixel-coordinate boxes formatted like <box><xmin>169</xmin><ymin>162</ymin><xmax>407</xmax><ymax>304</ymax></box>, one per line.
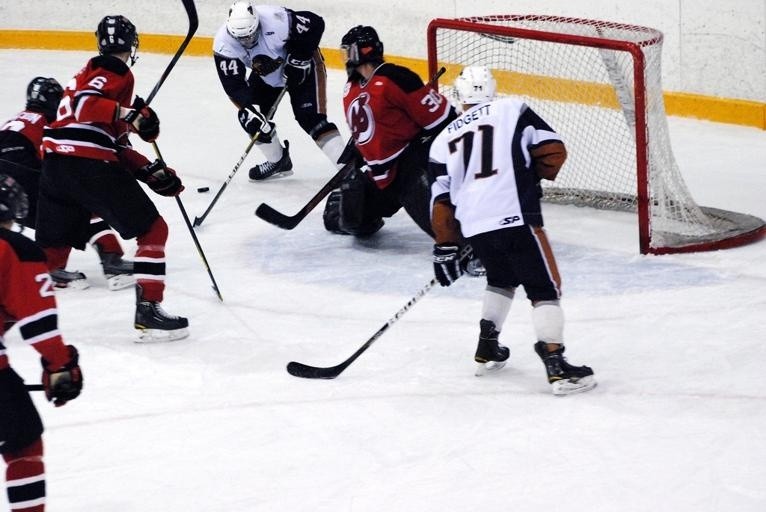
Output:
<box><xmin>40</xmin><ymin>345</ymin><xmax>81</xmax><ymax>405</ymax></box>
<box><xmin>433</xmin><ymin>244</ymin><xmax>463</xmax><ymax>287</ymax></box>
<box><xmin>239</xmin><ymin>104</ymin><xmax>273</xmax><ymax>143</ymax></box>
<box><xmin>124</xmin><ymin>96</ymin><xmax>160</xmax><ymax>142</ymax></box>
<box><xmin>137</xmin><ymin>160</ymin><xmax>184</xmax><ymax>196</ymax></box>
<box><xmin>283</xmin><ymin>52</ymin><xmax>312</xmax><ymax>86</ymax></box>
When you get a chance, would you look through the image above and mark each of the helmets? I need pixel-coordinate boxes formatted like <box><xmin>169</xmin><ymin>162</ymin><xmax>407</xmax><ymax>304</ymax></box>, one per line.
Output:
<box><xmin>26</xmin><ymin>77</ymin><xmax>63</xmax><ymax>123</ymax></box>
<box><xmin>1</xmin><ymin>174</ymin><xmax>28</xmax><ymax>226</ymax></box>
<box><xmin>453</xmin><ymin>65</ymin><xmax>496</xmax><ymax>114</ymax></box>
<box><xmin>227</xmin><ymin>2</ymin><xmax>260</xmax><ymax>47</ymax></box>
<box><xmin>96</xmin><ymin>16</ymin><xmax>138</xmax><ymax>69</ymax></box>
<box><xmin>340</xmin><ymin>26</ymin><xmax>383</xmax><ymax>83</ymax></box>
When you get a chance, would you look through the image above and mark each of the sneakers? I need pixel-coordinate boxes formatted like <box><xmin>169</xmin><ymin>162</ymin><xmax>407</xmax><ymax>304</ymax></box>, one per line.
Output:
<box><xmin>50</xmin><ymin>270</ymin><xmax>88</xmax><ymax>290</ymax></box>
<box><xmin>135</xmin><ymin>302</ymin><xmax>188</xmax><ymax>330</ymax></box>
<box><xmin>249</xmin><ymin>148</ymin><xmax>292</xmax><ymax>179</ymax></box>
<box><xmin>99</xmin><ymin>253</ymin><xmax>135</xmax><ymax>278</ymax></box>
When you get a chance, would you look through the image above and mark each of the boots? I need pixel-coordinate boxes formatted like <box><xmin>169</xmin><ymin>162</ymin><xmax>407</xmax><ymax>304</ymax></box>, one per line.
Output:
<box><xmin>475</xmin><ymin>319</ymin><xmax>510</xmax><ymax>362</ymax></box>
<box><xmin>534</xmin><ymin>342</ymin><xmax>593</xmax><ymax>383</ymax></box>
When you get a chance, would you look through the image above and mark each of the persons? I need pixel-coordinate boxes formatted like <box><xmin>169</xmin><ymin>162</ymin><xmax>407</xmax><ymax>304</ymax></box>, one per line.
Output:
<box><xmin>323</xmin><ymin>25</ymin><xmax>488</xmax><ymax>275</ymax></box>
<box><xmin>0</xmin><ymin>174</ymin><xmax>83</xmax><ymax>512</ymax></box>
<box><xmin>212</xmin><ymin>1</ymin><xmax>346</xmax><ymax>182</ymax></box>
<box><xmin>429</xmin><ymin>64</ymin><xmax>596</xmax><ymax>397</ymax></box>
<box><xmin>34</xmin><ymin>14</ymin><xmax>189</xmax><ymax>343</ymax></box>
<box><xmin>1</xmin><ymin>77</ymin><xmax>136</xmax><ymax>290</ymax></box>
